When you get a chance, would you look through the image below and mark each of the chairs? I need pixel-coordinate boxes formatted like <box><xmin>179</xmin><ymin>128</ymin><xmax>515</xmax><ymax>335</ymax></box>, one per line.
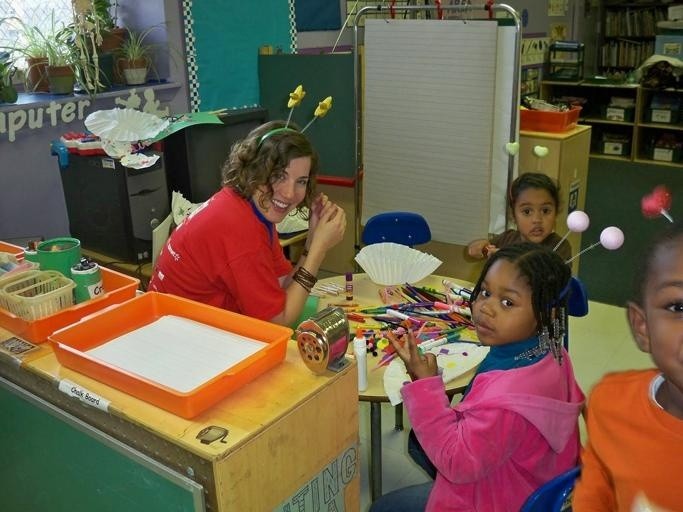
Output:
<box><xmin>362</xmin><ymin>212</ymin><xmax>431</xmax><ymax>250</ymax></box>
<box><xmin>519</xmin><ymin>464</ymin><xmax>581</xmax><ymax>512</ymax></box>
<box><xmin>561</xmin><ymin>276</ymin><xmax>588</xmax><ymax>352</ymax></box>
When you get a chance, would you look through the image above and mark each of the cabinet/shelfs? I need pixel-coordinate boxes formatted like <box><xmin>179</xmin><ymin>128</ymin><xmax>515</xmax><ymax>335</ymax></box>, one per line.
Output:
<box><xmin>517</xmin><ymin>125</ymin><xmax>591</xmax><ymax>278</ymax></box>
<box><xmin>540</xmin><ymin>0</ymin><xmax>683</xmax><ymax>167</ymax></box>
<box><xmin>0</xmin><ymin>289</ymin><xmax>360</xmax><ymax>512</ymax></box>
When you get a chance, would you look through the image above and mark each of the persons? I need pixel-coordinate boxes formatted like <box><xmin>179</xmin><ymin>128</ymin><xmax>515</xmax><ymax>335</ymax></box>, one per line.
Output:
<box><xmin>467</xmin><ymin>172</ymin><xmax>572</xmax><ymax>273</ymax></box>
<box><xmin>146</xmin><ymin>118</ymin><xmax>347</xmax><ymax>327</ymax></box>
<box><xmin>568</xmin><ymin>220</ymin><xmax>682</xmax><ymax>510</ymax></box>
<box><xmin>386</xmin><ymin>242</ymin><xmax>585</xmax><ymax>511</ymax></box>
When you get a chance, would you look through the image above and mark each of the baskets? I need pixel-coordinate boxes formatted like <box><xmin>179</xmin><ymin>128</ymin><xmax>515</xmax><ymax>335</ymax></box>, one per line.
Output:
<box><xmin>1</xmin><ymin>270</ymin><xmax>78</xmax><ymax>322</ymax></box>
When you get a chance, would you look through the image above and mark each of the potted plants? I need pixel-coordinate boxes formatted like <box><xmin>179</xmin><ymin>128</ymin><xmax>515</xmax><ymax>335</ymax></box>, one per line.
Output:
<box><xmin>0</xmin><ymin>0</ymin><xmax>187</xmax><ymax>94</ymax></box>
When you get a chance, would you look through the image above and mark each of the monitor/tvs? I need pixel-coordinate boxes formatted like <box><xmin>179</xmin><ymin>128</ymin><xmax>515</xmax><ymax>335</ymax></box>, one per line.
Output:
<box><xmin>161</xmin><ymin>107</ymin><xmax>269</xmax><ymax>212</ymax></box>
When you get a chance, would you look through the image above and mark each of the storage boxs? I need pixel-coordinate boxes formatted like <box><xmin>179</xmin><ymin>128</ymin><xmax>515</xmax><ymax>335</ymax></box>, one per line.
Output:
<box><xmin>520</xmin><ymin>104</ymin><xmax>582</xmax><ymax>133</ymax></box>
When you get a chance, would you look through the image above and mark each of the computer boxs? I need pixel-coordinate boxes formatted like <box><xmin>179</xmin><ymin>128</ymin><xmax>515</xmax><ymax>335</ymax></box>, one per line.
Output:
<box><xmin>58</xmin><ymin>148</ymin><xmax>172</xmax><ymax>266</ymax></box>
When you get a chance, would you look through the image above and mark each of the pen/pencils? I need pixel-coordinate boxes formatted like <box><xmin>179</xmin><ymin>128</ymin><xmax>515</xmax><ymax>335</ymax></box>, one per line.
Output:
<box><xmin>310</xmin><ymin>279</ymin><xmax>473</xmax><ymax>369</ymax></box>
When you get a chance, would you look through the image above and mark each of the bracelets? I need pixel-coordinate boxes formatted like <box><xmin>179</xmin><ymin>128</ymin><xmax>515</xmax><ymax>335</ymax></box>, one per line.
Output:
<box><xmin>292</xmin><ymin>266</ymin><xmax>318</xmax><ymax>294</ymax></box>
<box><xmin>301</xmin><ymin>249</ymin><xmax>308</xmax><ymax>255</ymax></box>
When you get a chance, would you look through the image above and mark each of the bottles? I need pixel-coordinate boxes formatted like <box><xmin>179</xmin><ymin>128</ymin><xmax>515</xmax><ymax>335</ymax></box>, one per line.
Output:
<box><xmin>36</xmin><ymin>238</ymin><xmax>81</xmax><ymax>280</ymax></box>
<box><xmin>23</xmin><ymin>245</ymin><xmax>38</xmax><ymax>263</ymax></box>
<box><xmin>354</xmin><ymin>326</ymin><xmax>368</xmax><ymax>394</ymax></box>
<box><xmin>71</xmin><ymin>262</ymin><xmax>103</xmax><ymax>305</ymax></box>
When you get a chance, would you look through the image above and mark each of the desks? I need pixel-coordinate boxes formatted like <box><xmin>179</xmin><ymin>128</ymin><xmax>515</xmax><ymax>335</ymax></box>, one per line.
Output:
<box><xmin>80</xmin><ymin>231</ymin><xmax>310</xmax><ymax>290</ymax></box>
<box><xmin>310</xmin><ymin>273</ymin><xmax>491</xmax><ymax>501</ymax></box>
